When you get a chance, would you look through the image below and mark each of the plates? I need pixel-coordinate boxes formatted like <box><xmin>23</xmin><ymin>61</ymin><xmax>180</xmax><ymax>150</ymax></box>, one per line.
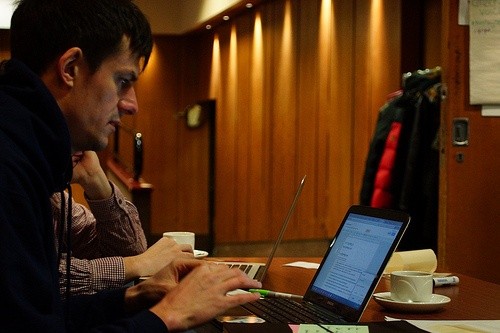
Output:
<box><xmin>373</xmin><ymin>292</ymin><xmax>451</xmax><ymax>312</ymax></box>
<box><xmin>192</xmin><ymin>250</ymin><xmax>209</xmax><ymax>258</ymax></box>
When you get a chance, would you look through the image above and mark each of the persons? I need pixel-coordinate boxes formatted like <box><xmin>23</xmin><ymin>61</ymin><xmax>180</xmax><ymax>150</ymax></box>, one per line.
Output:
<box><xmin>1</xmin><ymin>29</ymin><xmax>195</xmax><ymax>299</ymax></box>
<box><xmin>1</xmin><ymin>0</ymin><xmax>262</xmax><ymax>333</ymax></box>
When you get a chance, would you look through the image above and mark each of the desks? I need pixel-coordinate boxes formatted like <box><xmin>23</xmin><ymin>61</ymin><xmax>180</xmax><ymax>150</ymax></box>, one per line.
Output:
<box><xmin>202</xmin><ymin>257</ymin><xmax>500</xmax><ymax>333</ymax></box>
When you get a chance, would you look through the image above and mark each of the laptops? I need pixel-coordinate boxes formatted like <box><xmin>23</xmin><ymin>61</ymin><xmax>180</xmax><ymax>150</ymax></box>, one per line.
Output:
<box><xmin>137</xmin><ymin>174</ymin><xmax>307</xmax><ymax>285</ymax></box>
<box><xmin>215</xmin><ymin>205</ymin><xmax>412</xmax><ymax>325</ymax></box>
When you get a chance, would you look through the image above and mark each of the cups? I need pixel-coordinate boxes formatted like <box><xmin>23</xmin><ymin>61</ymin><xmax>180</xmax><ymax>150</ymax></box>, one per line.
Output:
<box><xmin>391</xmin><ymin>272</ymin><xmax>432</xmax><ymax>302</ymax></box>
<box><xmin>163</xmin><ymin>232</ymin><xmax>195</xmax><ymax>253</ymax></box>
